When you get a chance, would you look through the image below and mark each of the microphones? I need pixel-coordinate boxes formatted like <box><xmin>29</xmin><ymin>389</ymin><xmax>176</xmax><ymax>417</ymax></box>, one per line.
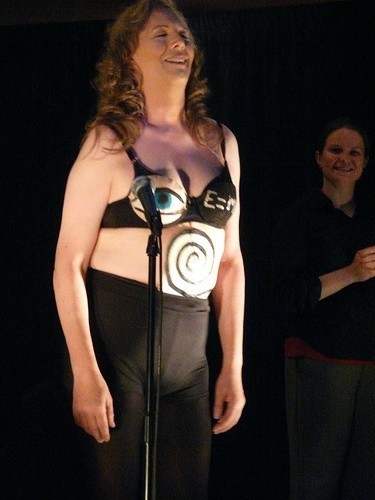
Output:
<box><xmin>131</xmin><ymin>175</ymin><xmax>164</xmax><ymax>237</ymax></box>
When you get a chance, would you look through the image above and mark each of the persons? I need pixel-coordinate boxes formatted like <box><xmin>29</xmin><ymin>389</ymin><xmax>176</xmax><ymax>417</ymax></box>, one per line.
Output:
<box><xmin>50</xmin><ymin>0</ymin><xmax>247</xmax><ymax>499</ymax></box>
<box><xmin>265</xmin><ymin>119</ymin><xmax>375</xmax><ymax>499</ymax></box>
